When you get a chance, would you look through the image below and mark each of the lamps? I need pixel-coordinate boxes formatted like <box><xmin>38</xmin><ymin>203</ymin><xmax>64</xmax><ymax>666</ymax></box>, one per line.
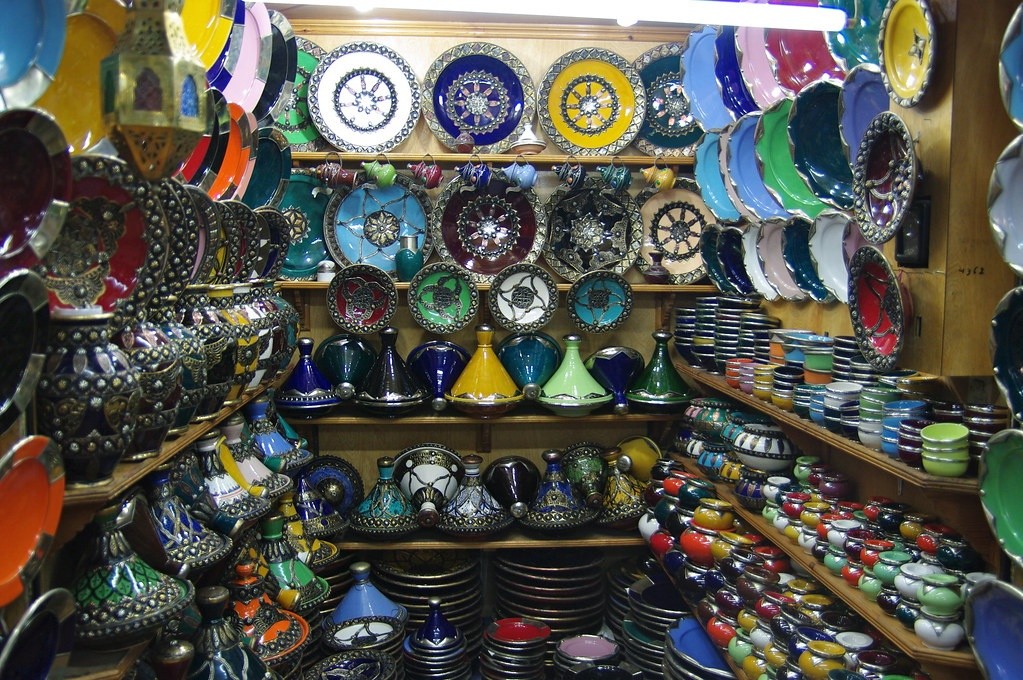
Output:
<box><xmin>276</xmin><ymin>0</ymin><xmax>848</xmax><ymax>34</ymax></box>
<box><xmin>894</xmin><ymin>137</ymin><xmax>934</xmax><ymax>272</ymax></box>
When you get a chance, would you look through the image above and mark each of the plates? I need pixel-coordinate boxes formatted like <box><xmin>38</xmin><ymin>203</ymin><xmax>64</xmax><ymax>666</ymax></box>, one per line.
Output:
<box><xmin>998</xmin><ymin>2</ymin><xmax>1022</xmax><ymax>133</ymax></box>
<box><xmin>694</xmin><ymin>63</ymin><xmax>918</xmax><ymax>373</ymax></box>
<box><xmin>1</xmin><ymin>268</ymin><xmax>76</xmax><ymax>680</ymax></box>
<box><xmin>679</xmin><ymin>0</ymin><xmax>938</xmax><ymax>135</ymax></box>
<box><xmin>0</xmin><ymin>110</ymin><xmax>290</xmax><ymax>309</ymax></box>
<box><xmin>273</xmin><ymin>175</ymin><xmax>718</xmax><ymax>284</ymax></box>
<box><xmin>964</xmin><ymin>578</ymin><xmax>1023</xmax><ymax>680</ymax></box>
<box><xmin>0</xmin><ymin>0</ymin><xmax>298</xmax><ymax>209</ymax></box>
<box><xmin>985</xmin><ymin>135</ymin><xmax>1022</xmax><ymax>277</ymax></box>
<box><xmin>273</xmin><ymin>36</ymin><xmax>703</xmax><ymax>156</ymax></box>
<box><xmin>303</xmin><ymin>547</ymin><xmax>738</xmax><ymax>680</ymax></box>
<box><xmin>979</xmin><ymin>430</ymin><xmax>1022</xmax><ymax>568</ymax></box>
<box><xmin>325</xmin><ymin>264</ymin><xmax>632</xmax><ymax>332</ymax></box>
<box><xmin>990</xmin><ymin>285</ymin><xmax>1023</xmax><ymax>426</ymax></box>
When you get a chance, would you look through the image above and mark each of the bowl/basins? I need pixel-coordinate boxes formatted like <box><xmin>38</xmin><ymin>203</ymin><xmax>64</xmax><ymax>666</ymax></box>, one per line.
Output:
<box><xmin>672</xmin><ymin>297</ymin><xmax>1012</xmax><ymax>478</ymax></box>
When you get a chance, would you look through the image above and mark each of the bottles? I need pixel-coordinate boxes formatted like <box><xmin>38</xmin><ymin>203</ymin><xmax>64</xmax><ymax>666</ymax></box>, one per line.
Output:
<box><xmin>275</xmin><ymin>324</ymin><xmax>705</xmax><ymax>419</ymax></box>
<box><xmin>67</xmin><ymin>386</ymin><xmax>339</xmax><ymax>680</ymax></box>
<box><xmin>639</xmin><ymin>396</ymin><xmax>997</xmax><ymax>680</ymax></box>
<box><xmin>346</xmin><ymin>434</ymin><xmax>664</xmax><ymax>543</ymax></box>
<box><xmin>28</xmin><ymin>277</ymin><xmax>299</xmax><ymax>490</ymax></box>
<box><xmin>645</xmin><ymin>253</ymin><xmax>670</xmax><ymax>286</ymax></box>
<box><xmin>321</xmin><ymin>560</ymin><xmax>408</xmax><ymax>632</ymax></box>
<box><xmin>413</xmin><ymin>597</ymin><xmax>463</xmax><ymax>650</ymax></box>
<box><xmin>396</xmin><ymin>235</ymin><xmax>423</xmax><ymax>283</ymax></box>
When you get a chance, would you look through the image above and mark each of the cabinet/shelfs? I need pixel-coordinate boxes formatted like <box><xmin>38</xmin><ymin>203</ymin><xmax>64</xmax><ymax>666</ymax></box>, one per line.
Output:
<box><xmin>675</xmin><ymin>361</ymin><xmax>1008</xmax><ymax>667</ymax></box>
<box><xmin>268</xmin><ymin>148</ymin><xmax>719</xmax><ymax>554</ymax></box>
<box><xmin>45</xmin><ymin>364</ymin><xmax>294</xmax><ymax>680</ymax></box>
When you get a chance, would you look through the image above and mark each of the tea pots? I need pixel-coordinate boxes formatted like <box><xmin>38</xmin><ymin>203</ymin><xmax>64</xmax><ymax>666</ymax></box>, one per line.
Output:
<box><xmin>310</xmin><ymin>150</ymin><xmax>678</xmax><ymax>194</ymax></box>
